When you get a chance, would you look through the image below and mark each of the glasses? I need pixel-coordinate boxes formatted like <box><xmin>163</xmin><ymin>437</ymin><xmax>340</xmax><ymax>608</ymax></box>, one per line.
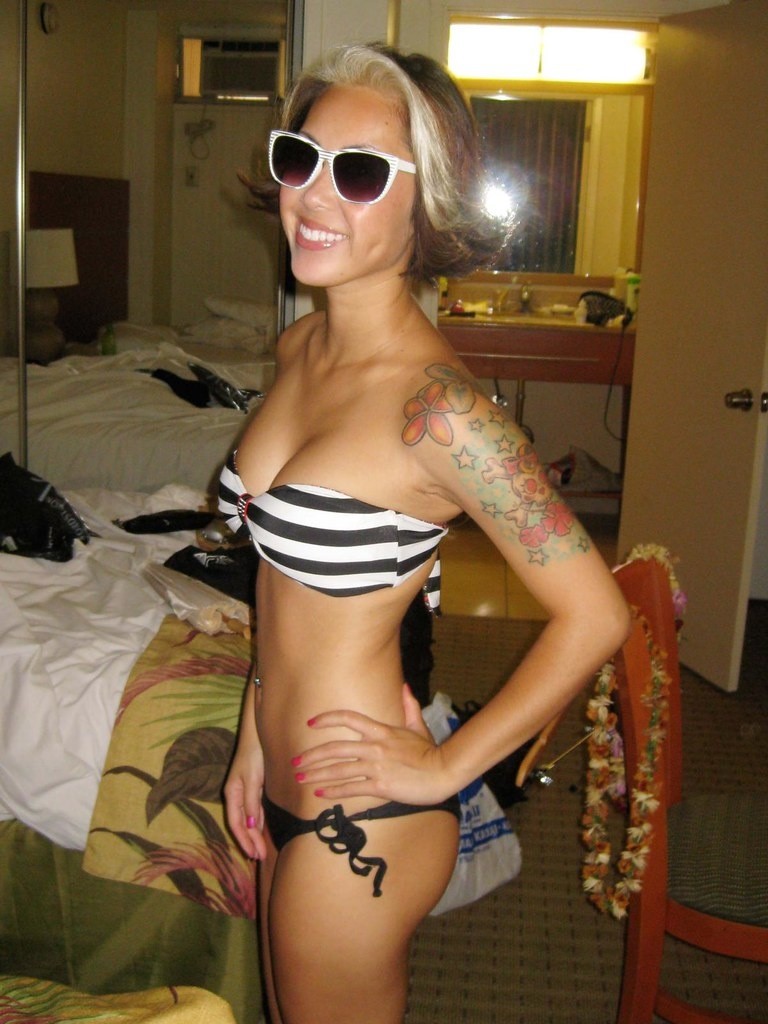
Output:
<box><xmin>268</xmin><ymin>130</ymin><xmax>416</xmax><ymax>204</ymax></box>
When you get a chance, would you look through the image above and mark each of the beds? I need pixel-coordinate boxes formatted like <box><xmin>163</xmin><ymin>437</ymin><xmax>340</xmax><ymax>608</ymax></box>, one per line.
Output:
<box><xmin>0</xmin><ymin>321</ymin><xmax>277</xmax><ymax>1024</ymax></box>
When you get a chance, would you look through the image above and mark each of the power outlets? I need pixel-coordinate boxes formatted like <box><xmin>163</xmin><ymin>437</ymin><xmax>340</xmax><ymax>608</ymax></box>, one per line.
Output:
<box><xmin>186</xmin><ymin>166</ymin><xmax>199</xmax><ymax>185</ymax></box>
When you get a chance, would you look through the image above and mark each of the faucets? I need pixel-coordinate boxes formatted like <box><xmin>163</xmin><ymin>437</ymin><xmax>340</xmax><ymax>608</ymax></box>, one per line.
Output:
<box><xmin>516</xmin><ymin>286</ymin><xmax>535</xmax><ymax>314</ymax></box>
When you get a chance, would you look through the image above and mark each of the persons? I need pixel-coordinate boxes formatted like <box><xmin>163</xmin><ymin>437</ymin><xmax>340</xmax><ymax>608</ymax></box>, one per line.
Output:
<box><xmin>209</xmin><ymin>43</ymin><xmax>633</xmax><ymax>1023</ymax></box>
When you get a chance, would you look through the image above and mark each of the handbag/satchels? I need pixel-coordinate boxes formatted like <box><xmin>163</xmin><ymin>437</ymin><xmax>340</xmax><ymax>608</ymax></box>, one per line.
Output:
<box><xmin>418</xmin><ymin>692</ymin><xmax>523</xmax><ymax>916</ymax></box>
<box><xmin>539</xmin><ymin>445</ymin><xmax>621</xmax><ymax>493</ymax></box>
<box><xmin>578</xmin><ymin>291</ymin><xmax>624</xmax><ymax>326</ymax></box>
<box><xmin>454</xmin><ymin>699</ymin><xmax>540</xmax><ymax>806</ymax></box>
<box><xmin>0</xmin><ymin>452</ymin><xmax>90</xmax><ymax>562</ymax></box>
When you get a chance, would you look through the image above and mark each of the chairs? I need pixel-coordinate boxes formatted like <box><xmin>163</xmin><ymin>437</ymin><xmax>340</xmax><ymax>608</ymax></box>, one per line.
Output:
<box><xmin>610</xmin><ymin>558</ymin><xmax>768</xmax><ymax>1024</ymax></box>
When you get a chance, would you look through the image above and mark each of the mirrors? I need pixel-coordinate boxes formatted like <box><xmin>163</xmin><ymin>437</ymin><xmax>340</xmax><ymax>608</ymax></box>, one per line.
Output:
<box><xmin>446</xmin><ymin>10</ymin><xmax>660</xmax><ymax>285</ymax></box>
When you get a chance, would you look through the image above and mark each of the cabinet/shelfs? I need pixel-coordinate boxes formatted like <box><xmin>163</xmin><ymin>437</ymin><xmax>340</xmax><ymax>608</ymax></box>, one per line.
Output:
<box><xmin>438</xmin><ymin>309</ymin><xmax>637</xmax><ymax>622</ymax></box>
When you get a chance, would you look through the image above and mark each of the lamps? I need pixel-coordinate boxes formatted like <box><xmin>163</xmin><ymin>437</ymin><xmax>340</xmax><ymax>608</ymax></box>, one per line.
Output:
<box><xmin>7</xmin><ymin>228</ymin><xmax>80</xmax><ymax>364</ymax></box>
